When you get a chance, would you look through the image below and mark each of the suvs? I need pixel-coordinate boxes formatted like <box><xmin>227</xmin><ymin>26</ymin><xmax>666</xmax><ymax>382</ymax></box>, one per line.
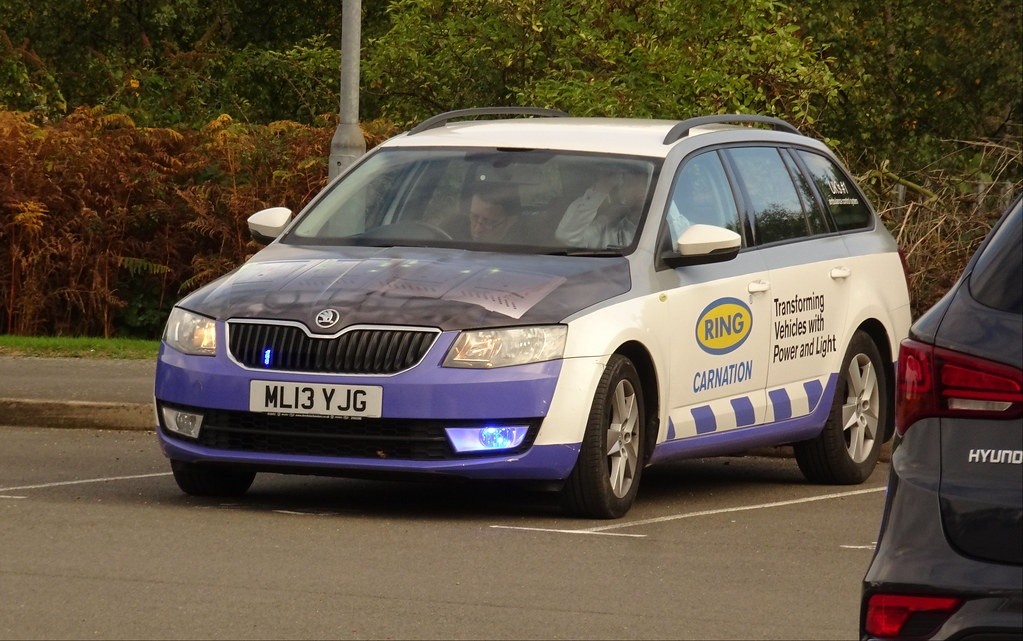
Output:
<box><xmin>861</xmin><ymin>183</ymin><xmax>1023</xmax><ymax>641</ymax></box>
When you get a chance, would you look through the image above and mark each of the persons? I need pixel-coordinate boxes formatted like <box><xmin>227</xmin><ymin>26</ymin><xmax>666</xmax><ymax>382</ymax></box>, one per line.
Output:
<box><xmin>555</xmin><ymin>163</ymin><xmax>692</xmax><ymax>254</ymax></box>
<box><xmin>449</xmin><ymin>179</ymin><xmax>539</xmax><ymax>246</ymax></box>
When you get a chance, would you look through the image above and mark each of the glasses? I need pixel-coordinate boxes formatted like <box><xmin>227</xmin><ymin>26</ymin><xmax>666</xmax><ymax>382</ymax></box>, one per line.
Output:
<box><xmin>466</xmin><ymin>211</ymin><xmax>506</xmax><ymax>230</ymax></box>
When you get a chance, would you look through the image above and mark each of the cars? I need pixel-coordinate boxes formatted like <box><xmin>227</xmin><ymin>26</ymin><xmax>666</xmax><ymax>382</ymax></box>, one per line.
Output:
<box><xmin>154</xmin><ymin>114</ymin><xmax>913</xmax><ymax>521</ymax></box>
